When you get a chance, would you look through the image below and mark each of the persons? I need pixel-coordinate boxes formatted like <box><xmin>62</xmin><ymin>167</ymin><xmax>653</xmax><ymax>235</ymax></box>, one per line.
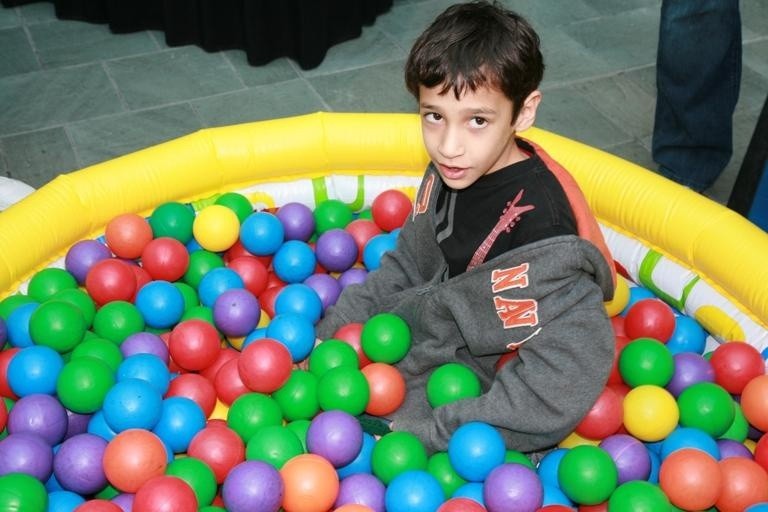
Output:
<box><xmin>651</xmin><ymin>0</ymin><xmax>744</xmax><ymax>191</ymax></box>
<box><xmin>303</xmin><ymin>0</ymin><xmax>619</xmax><ymax>457</ymax></box>
